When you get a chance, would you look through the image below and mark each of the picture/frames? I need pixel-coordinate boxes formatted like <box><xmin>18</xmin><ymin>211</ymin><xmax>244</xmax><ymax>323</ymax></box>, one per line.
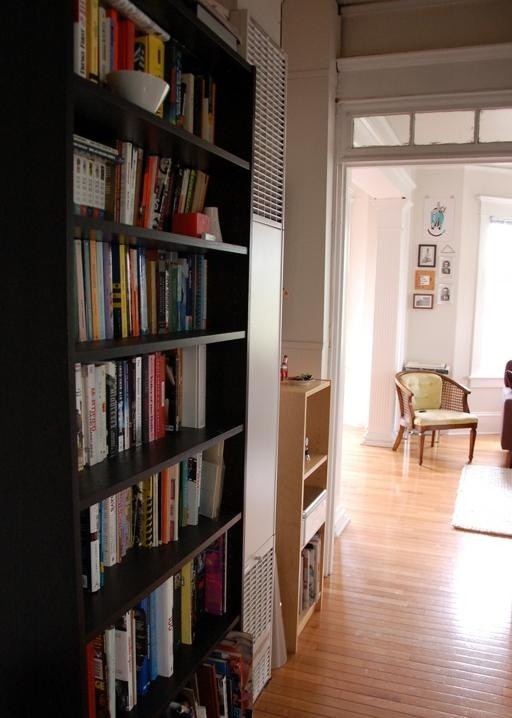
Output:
<box><xmin>413</xmin><ymin>244</ymin><xmax>454</xmax><ymax>309</ymax></box>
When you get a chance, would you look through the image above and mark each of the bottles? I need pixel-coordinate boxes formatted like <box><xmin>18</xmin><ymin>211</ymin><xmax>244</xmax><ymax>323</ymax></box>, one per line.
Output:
<box><xmin>281</xmin><ymin>354</ymin><xmax>288</xmax><ymax>382</ymax></box>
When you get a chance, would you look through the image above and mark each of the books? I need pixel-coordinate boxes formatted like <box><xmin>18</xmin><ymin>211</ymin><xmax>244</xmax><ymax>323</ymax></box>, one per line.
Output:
<box><xmin>81</xmin><ymin>439</ymin><xmax>226</xmax><ymax>593</ymax></box>
<box><xmin>75</xmin><ymin>344</ymin><xmax>205</xmax><ymax>472</ymax></box>
<box><xmin>73</xmin><ymin>1</ymin><xmax>242</xmax><ymax>145</ymax></box>
<box><xmin>86</xmin><ymin>535</ymin><xmax>252</xmax><ymax>717</ymax></box>
<box><xmin>299</xmin><ymin>534</ymin><xmax>324</xmax><ymax>617</ymax></box>
<box><xmin>72</xmin><ymin>226</ymin><xmax>207</xmax><ymax>342</ymax></box>
<box><xmin>73</xmin><ymin>134</ymin><xmax>222</xmax><ymax>242</ymax></box>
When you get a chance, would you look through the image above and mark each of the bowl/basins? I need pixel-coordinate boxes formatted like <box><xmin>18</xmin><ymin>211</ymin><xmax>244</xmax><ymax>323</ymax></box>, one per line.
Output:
<box><xmin>104</xmin><ymin>70</ymin><xmax>169</xmax><ymax>114</ymax></box>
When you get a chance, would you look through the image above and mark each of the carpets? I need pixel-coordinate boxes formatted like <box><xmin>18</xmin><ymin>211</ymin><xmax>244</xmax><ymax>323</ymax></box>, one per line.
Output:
<box><xmin>451</xmin><ymin>463</ymin><xmax>512</xmax><ymax>541</ymax></box>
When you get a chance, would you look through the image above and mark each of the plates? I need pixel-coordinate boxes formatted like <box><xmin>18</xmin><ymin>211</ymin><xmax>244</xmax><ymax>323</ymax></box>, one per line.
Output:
<box><xmin>286</xmin><ymin>379</ymin><xmax>315</xmax><ymax>386</ymax></box>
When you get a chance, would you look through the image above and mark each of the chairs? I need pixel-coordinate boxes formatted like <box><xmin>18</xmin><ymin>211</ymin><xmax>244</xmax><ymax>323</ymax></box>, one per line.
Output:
<box><xmin>391</xmin><ymin>369</ymin><xmax>479</xmax><ymax>466</ymax></box>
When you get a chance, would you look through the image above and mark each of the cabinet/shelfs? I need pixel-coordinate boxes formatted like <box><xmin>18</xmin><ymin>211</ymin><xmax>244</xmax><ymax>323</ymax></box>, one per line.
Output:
<box><xmin>279</xmin><ymin>377</ymin><xmax>334</xmax><ymax>656</ymax></box>
<box><xmin>1</xmin><ymin>1</ymin><xmax>259</xmax><ymax>718</ymax></box>
